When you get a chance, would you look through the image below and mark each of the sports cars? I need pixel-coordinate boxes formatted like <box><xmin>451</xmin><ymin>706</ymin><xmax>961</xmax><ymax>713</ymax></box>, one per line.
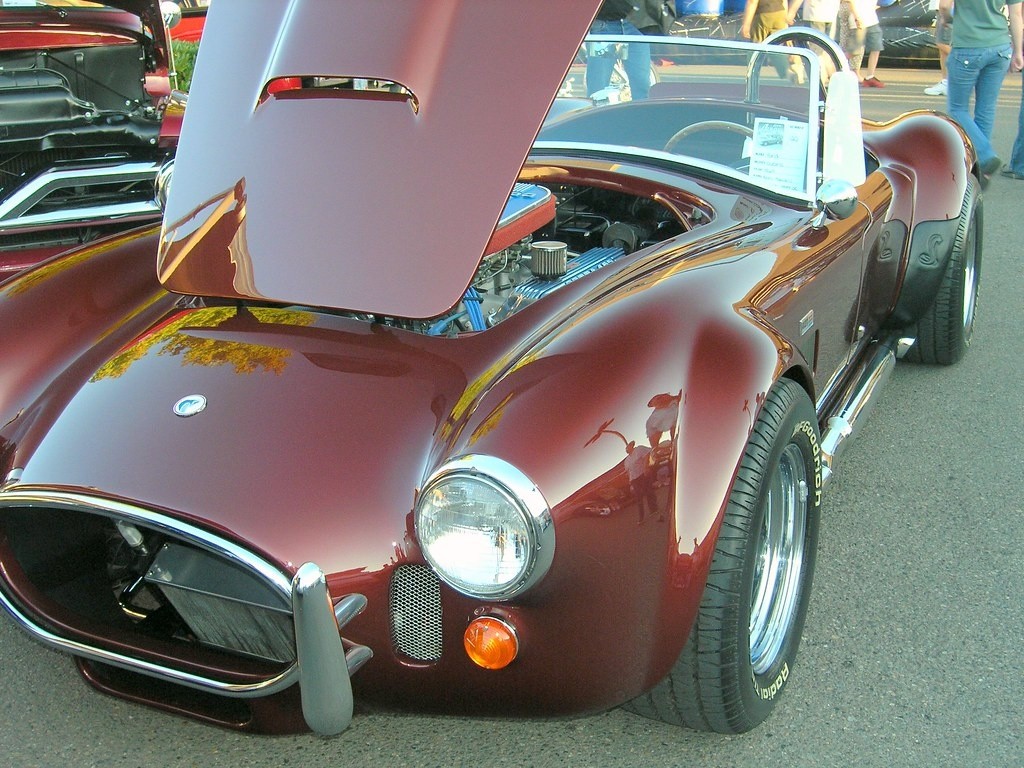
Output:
<box><xmin>0</xmin><ymin>0</ymin><xmax>989</xmax><ymax>733</ymax></box>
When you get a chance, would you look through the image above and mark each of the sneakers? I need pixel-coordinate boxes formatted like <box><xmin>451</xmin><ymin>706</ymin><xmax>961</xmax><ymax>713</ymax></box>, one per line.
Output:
<box><xmin>864</xmin><ymin>77</ymin><xmax>886</xmax><ymax>88</ymax></box>
<box><xmin>924</xmin><ymin>81</ymin><xmax>948</xmax><ymax>96</ymax></box>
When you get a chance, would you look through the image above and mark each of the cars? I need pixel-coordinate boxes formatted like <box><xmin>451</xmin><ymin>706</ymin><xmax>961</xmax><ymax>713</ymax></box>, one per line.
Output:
<box><xmin>0</xmin><ymin>0</ymin><xmax>188</xmax><ymax>283</ymax></box>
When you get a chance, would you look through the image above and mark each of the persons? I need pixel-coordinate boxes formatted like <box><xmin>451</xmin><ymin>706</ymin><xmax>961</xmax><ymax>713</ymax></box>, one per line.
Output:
<box><xmin>586</xmin><ymin>0</ymin><xmax>651</xmax><ymax>106</ymax></box>
<box><xmin>939</xmin><ymin>0</ymin><xmax>1024</xmax><ymax>190</ymax></box>
<box><xmin>838</xmin><ymin>0</ymin><xmax>864</xmax><ymax>73</ymax></box>
<box><xmin>852</xmin><ymin>0</ymin><xmax>886</xmax><ymax>88</ymax></box>
<box><xmin>786</xmin><ymin>0</ymin><xmax>840</xmax><ymax>88</ymax></box>
<box><xmin>925</xmin><ymin>0</ymin><xmax>954</xmax><ymax>96</ymax></box>
<box><xmin>998</xmin><ymin>71</ymin><xmax>1024</xmax><ymax>180</ymax></box>
<box><xmin>741</xmin><ymin>0</ymin><xmax>790</xmax><ymax>79</ymax></box>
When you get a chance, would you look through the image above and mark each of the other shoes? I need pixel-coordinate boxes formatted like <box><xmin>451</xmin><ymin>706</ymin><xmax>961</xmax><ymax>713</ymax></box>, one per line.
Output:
<box><xmin>980</xmin><ymin>156</ymin><xmax>1001</xmax><ymax>174</ymax></box>
<box><xmin>1001</xmin><ymin>163</ymin><xmax>1024</xmax><ymax>180</ymax></box>
<box><xmin>785</xmin><ymin>73</ymin><xmax>799</xmax><ymax>86</ymax></box>
